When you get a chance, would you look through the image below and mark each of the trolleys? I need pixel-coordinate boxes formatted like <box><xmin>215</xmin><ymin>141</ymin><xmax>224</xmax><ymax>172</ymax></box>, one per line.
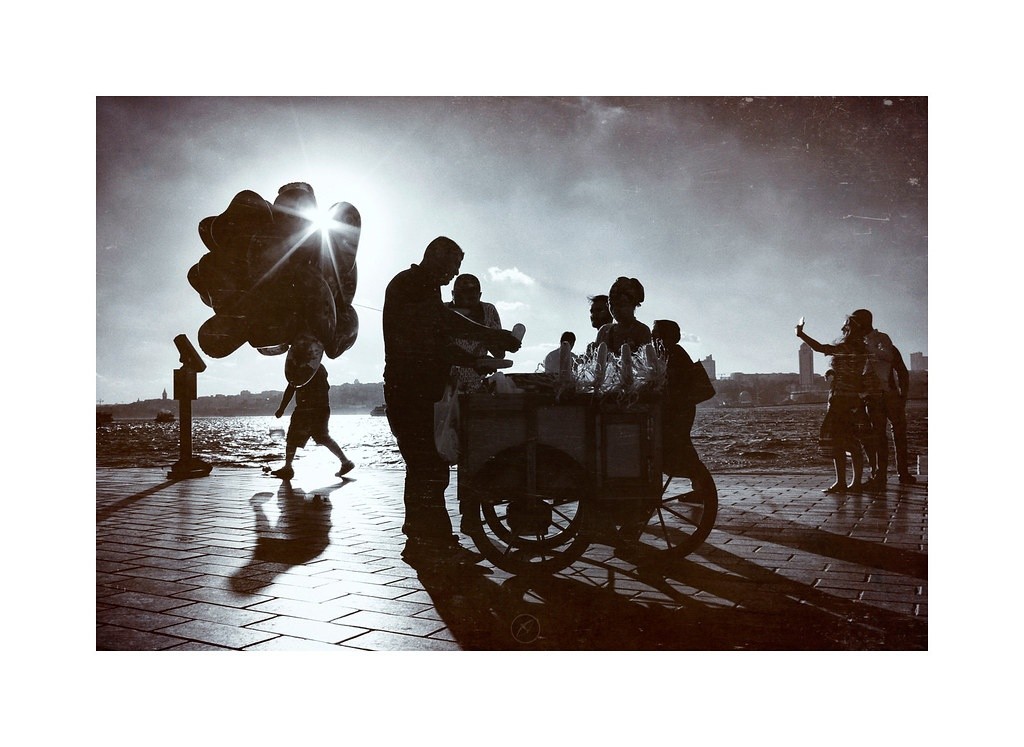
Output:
<box><xmin>457</xmin><ymin>373</ymin><xmax>719</xmax><ymax>580</ymax></box>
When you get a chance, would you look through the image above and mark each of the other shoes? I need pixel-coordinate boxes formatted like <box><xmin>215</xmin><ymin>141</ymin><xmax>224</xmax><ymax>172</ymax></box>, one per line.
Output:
<box><xmin>271</xmin><ymin>467</ymin><xmax>295</xmax><ymax>475</ymax></box>
<box><xmin>875</xmin><ymin>468</ymin><xmax>888</xmax><ymax>483</ymax></box>
<box><xmin>335</xmin><ymin>460</ymin><xmax>355</xmax><ymax>477</ymax></box>
<box><xmin>824</xmin><ymin>482</ymin><xmax>848</xmax><ymax>493</ymax></box>
<box><xmin>899</xmin><ymin>473</ymin><xmax>917</xmax><ymax>482</ymax></box>
<box><xmin>400</xmin><ymin>535</ymin><xmax>485</xmax><ymax>564</ymax></box>
<box><xmin>861</xmin><ymin>477</ymin><xmax>885</xmax><ymax>490</ymax></box>
<box><xmin>678</xmin><ymin>491</ymin><xmax>704</xmax><ymax>504</ymax></box>
<box><xmin>847</xmin><ymin>482</ymin><xmax>862</xmax><ymax>492</ymax></box>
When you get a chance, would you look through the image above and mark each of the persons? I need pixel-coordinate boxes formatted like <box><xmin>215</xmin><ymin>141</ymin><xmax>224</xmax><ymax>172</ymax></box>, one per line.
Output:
<box><xmin>545</xmin><ymin>331</ymin><xmax>580</xmax><ymax>374</ymax></box>
<box><xmin>597</xmin><ymin>277</ymin><xmax>649</xmax><ymax>359</ymax></box>
<box><xmin>795</xmin><ymin>309</ymin><xmax>917</xmax><ymax>494</ymax></box>
<box><xmin>587</xmin><ymin>295</ymin><xmax>614</xmax><ymax>329</ymax></box>
<box><xmin>440</xmin><ymin>274</ymin><xmax>505</xmax><ymax>536</ymax></box>
<box><xmin>653</xmin><ymin>320</ymin><xmax>707</xmax><ymax>503</ymax></box>
<box><xmin>270</xmin><ymin>365</ymin><xmax>355</xmax><ymax>479</ymax></box>
<box><xmin>383</xmin><ymin>235</ymin><xmax>522</xmax><ymax>560</ymax></box>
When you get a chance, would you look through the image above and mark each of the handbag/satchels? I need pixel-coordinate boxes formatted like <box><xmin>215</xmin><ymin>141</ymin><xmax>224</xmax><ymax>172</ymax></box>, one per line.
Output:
<box><xmin>693</xmin><ymin>360</ymin><xmax>716</xmax><ymax>404</ymax></box>
<box><xmin>433</xmin><ymin>378</ymin><xmax>461</xmax><ymax>464</ymax></box>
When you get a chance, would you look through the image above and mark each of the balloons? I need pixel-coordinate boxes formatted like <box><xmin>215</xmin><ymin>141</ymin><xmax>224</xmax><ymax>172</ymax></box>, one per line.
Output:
<box><xmin>186</xmin><ymin>182</ymin><xmax>360</xmax><ymax>387</ymax></box>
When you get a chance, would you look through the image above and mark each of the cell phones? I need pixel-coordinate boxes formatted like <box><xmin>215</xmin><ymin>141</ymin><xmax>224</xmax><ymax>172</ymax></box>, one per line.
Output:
<box><xmin>799</xmin><ymin>317</ymin><xmax>805</xmax><ymax>326</ymax></box>
